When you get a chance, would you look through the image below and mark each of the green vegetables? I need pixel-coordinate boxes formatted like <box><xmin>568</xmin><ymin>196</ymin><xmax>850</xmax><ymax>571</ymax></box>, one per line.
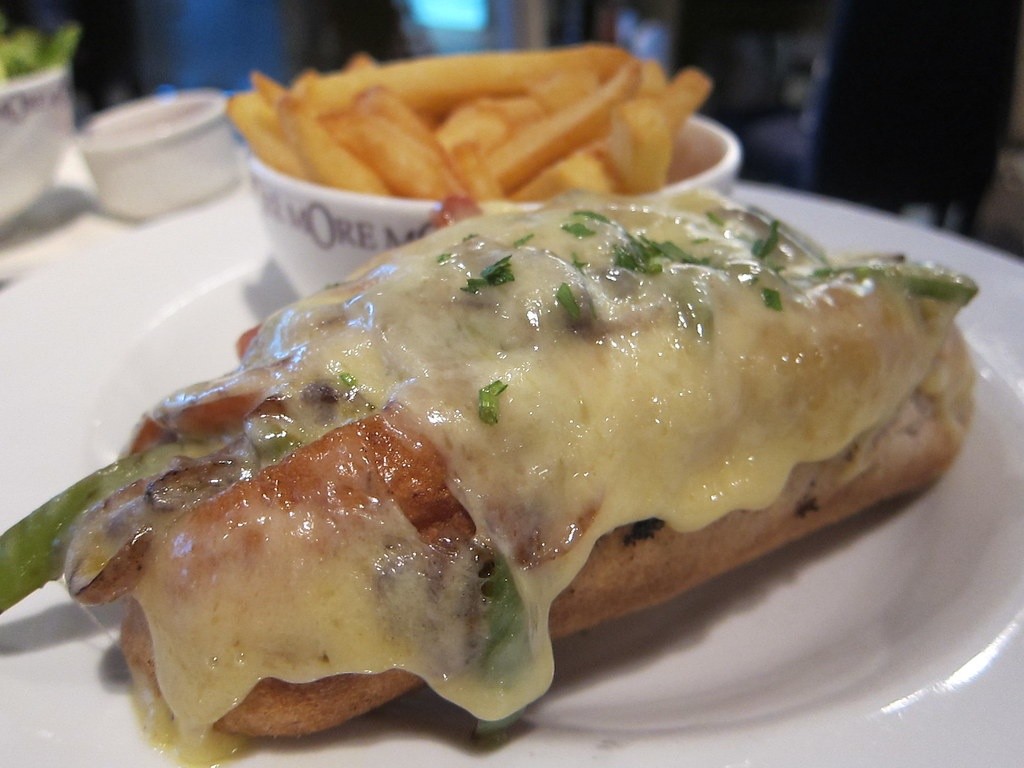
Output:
<box><xmin>0</xmin><ymin>23</ymin><xmax>80</xmax><ymax>80</ymax></box>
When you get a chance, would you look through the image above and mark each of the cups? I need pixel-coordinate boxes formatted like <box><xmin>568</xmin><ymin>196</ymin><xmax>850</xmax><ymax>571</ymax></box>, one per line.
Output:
<box><xmin>76</xmin><ymin>88</ymin><xmax>239</xmax><ymax>222</ymax></box>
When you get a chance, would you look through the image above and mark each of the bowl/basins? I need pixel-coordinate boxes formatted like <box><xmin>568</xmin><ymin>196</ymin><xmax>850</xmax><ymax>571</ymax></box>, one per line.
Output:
<box><xmin>0</xmin><ymin>68</ymin><xmax>76</xmax><ymax>229</ymax></box>
<box><xmin>239</xmin><ymin>111</ymin><xmax>743</xmax><ymax>298</ymax></box>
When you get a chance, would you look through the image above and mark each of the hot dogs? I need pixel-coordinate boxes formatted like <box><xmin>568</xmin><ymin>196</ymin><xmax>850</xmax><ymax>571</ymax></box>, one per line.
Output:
<box><xmin>0</xmin><ymin>185</ymin><xmax>983</xmax><ymax>739</ymax></box>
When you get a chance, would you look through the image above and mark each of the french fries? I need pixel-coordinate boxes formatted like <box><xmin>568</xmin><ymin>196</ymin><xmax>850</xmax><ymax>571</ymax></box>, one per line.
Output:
<box><xmin>226</xmin><ymin>45</ymin><xmax>712</xmax><ymax>203</ymax></box>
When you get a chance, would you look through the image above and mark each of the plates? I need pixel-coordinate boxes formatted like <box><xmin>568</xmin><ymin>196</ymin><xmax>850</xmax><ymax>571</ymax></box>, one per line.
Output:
<box><xmin>0</xmin><ymin>178</ymin><xmax>1023</xmax><ymax>767</ymax></box>
